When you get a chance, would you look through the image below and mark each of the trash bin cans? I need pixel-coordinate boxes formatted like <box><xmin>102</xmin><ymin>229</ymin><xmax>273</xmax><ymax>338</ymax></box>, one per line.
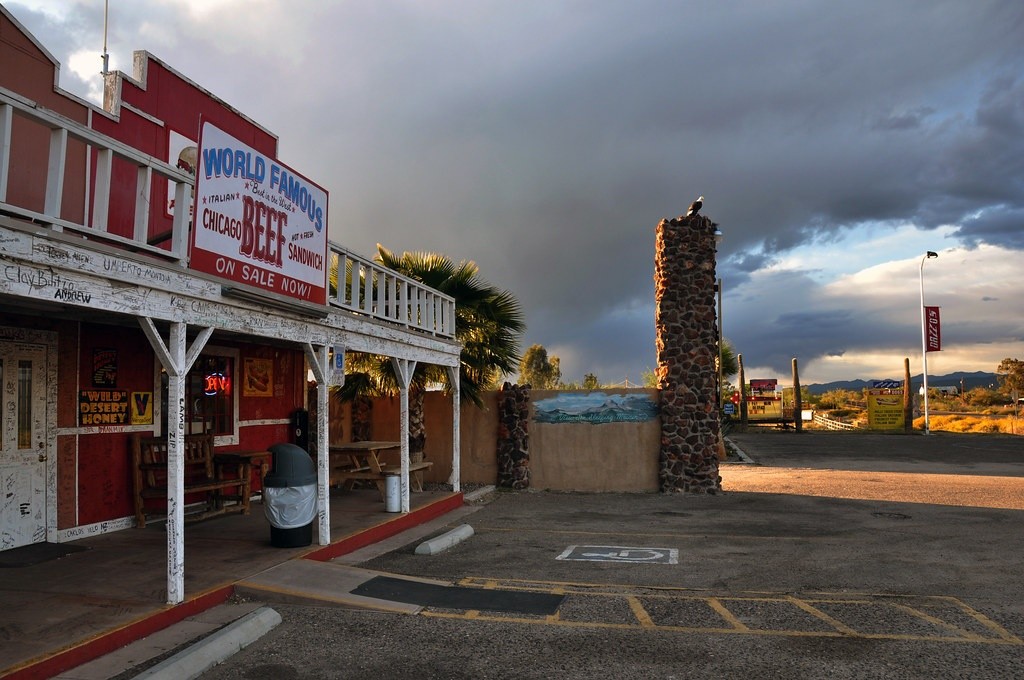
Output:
<box><xmin>263</xmin><ymin>444</ymin><xmax>318</xmax><ymax>548</ymax></box>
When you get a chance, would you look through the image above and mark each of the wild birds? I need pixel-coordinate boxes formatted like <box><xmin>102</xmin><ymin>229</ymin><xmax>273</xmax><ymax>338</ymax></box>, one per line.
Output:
<box><xmin>686</xmin><ymin>196</ymin><xmax>704</xmax><ymax>216</ymax></box>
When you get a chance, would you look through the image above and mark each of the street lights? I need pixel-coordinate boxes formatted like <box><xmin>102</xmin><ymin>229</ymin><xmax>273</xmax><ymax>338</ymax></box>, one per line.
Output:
<box><xmin>919</xmin><ymin>251</ymin><xmax>939</xmax><ymax>434</ymax></box>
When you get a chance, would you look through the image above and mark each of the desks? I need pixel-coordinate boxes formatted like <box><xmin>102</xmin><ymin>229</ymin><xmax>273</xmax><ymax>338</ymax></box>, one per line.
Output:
<box><xmin>215</xmin><ymin>449</ymin><xmax>271</xmax><ymax>508</ymax></box>
<box><xmin>333</xmin><ymin>437</ymin><xmax>412</xmax><ymax>498</ymax></box>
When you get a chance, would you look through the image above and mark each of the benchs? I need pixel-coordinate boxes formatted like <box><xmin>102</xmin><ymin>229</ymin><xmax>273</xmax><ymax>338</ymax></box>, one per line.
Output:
<box><xmin>382</xmin><ymin>461</ymin><xmax>435</xmax><ymax>502</ymax></box>
<box><xmin>127</xmin><ymin>430</ymin><xmax>253</xmax><ymax>525</ymax></box>
<box><xmin>332</xmin><ymin>462</ymin><xmax>389</xmax><ymax>492</ymax></box>
